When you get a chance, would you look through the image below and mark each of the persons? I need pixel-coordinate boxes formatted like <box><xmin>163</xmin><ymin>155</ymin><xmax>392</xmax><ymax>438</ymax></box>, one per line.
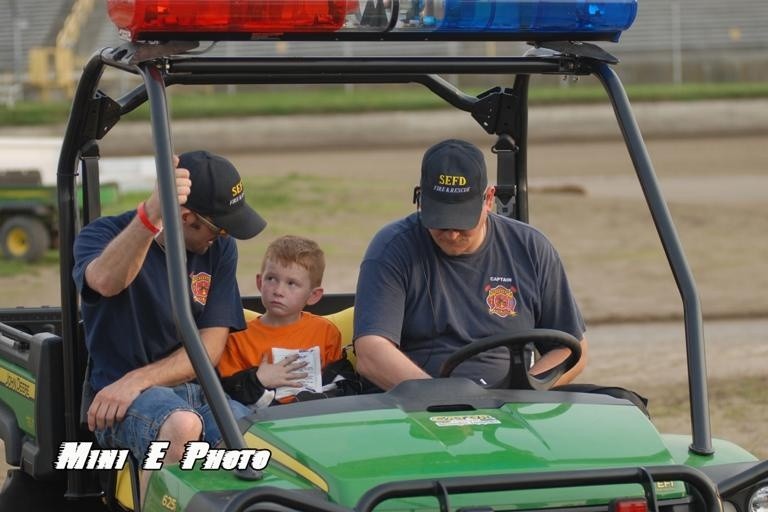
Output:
<box><xmin>216</xmin><ymin>235</ymin><xmax>366</xmax><ymax>406</ymax></box>
<box><xmin>352</xmin><ymin>138</ymin><xmax>588</xmax><ymax>391</ymax></box>
<box><xmin>71</xmin><ymin>149</ymin><xmax>267</xmax><ymax>512</ymax></box>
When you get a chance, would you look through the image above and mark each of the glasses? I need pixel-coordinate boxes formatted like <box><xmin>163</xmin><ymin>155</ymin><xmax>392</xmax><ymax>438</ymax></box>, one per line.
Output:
<box><xmin>191</xmin><ymin>210</ymin><xmax>233</xmax><ymax>242</ymax></box>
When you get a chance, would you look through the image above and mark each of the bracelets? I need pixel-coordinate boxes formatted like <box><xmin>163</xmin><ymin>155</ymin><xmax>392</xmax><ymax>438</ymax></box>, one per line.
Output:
<box><xmin>137</xmin><ymin>200</ymin><xmax>160</xmax><ymax>235</ymax></box>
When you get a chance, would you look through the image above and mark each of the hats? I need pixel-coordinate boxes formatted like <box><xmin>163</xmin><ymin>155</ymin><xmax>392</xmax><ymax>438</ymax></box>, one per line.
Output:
<box><xmin>415</xmin><ymin>138</ymin><xmax>488</xmax><ymax>232</ymax></box>
<box><xmin>173</xmin><ymin>149</ymin><xmax>268</xmax><ymax>239</ymax></box>
